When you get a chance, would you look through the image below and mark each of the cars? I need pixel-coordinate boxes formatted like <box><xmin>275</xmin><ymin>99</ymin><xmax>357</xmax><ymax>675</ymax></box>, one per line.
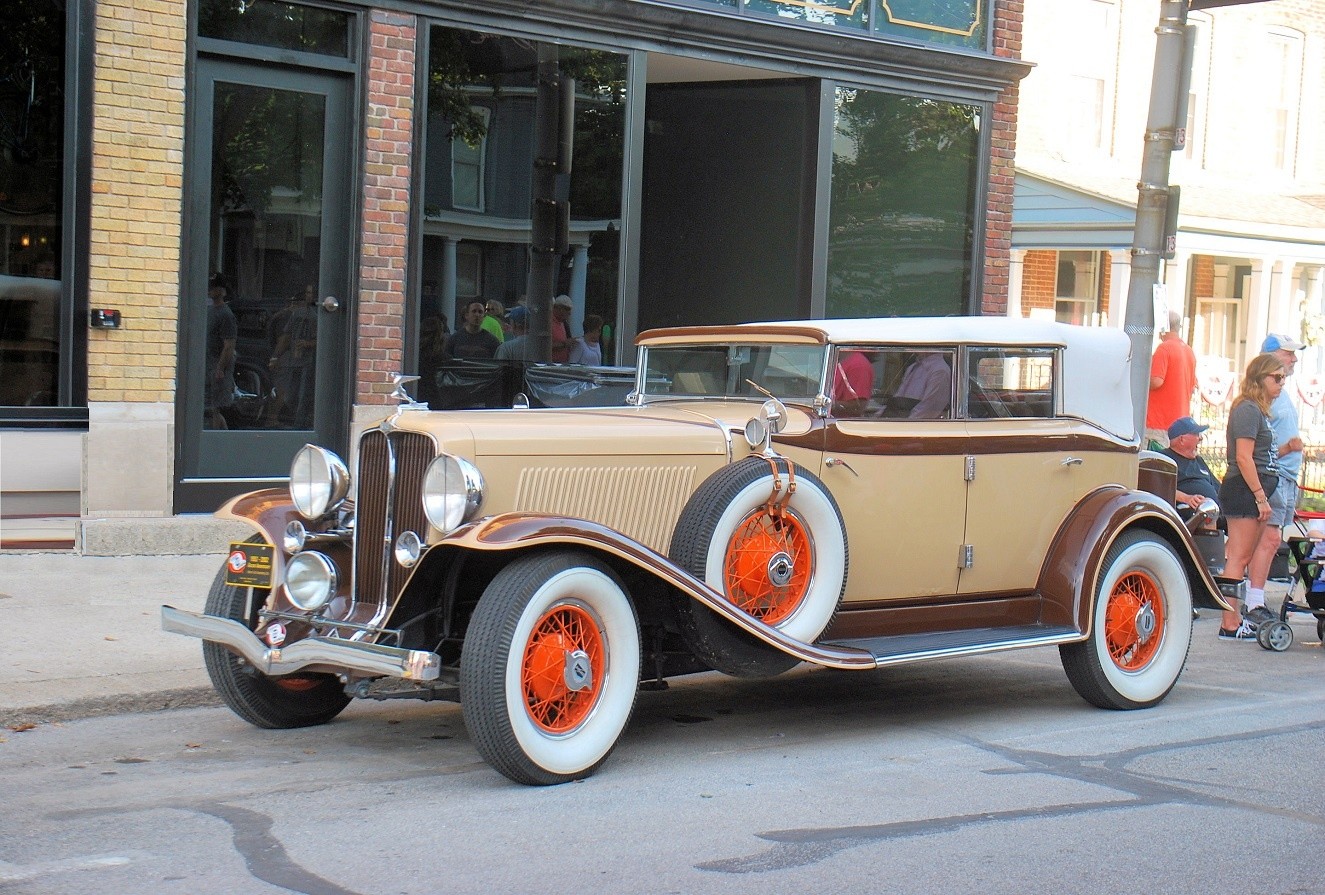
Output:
<box><xmin>161</xmin><ymin>317</ymin><xmax>1236</xmax><ymax>789</ymax></box>
<box><xmin>0</xmin><ymin>274</ymin><xmax>87</xmax><ymax>408</ymax></box>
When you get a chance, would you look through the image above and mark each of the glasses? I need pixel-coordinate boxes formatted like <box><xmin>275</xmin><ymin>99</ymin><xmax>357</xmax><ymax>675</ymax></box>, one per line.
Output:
<box><xmin>1268</xmin><ymin>374</ymin><xmax>1286</xmax><ymax>383</ymax></box>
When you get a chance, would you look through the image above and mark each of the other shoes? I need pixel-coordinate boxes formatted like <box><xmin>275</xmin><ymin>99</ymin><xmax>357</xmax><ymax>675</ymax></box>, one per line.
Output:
<box><xmin>1218</xmin><ymin>627</ymin><xmax>1257</xmax><ymax>642</ymax></box>
<box><xmin>1240</xmin><ymin>620</ymin><xmax>1257</xmax><ymax>633</ymax></box>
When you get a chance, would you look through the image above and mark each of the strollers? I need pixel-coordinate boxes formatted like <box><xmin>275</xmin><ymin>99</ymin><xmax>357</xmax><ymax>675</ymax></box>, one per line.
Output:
<box><xmin>1255</xmin><ymin>536</ymin><xmax>1325</xmax><ymax>652</ymax></box>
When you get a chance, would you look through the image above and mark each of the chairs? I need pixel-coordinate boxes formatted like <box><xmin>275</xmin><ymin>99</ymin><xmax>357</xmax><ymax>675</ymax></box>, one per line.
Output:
<box><xmin>1292</xmin><ymin>486</ymin><xmax>1325</xmax><ymax>537</ymax></box>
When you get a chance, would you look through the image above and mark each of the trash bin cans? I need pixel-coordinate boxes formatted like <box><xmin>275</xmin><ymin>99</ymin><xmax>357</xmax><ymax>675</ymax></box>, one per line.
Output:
<box><xmin>423</xmin><ymin>356</ymin><xmax>526</xmax><ymax>409</ymax></box>
<box><xmin>521</xmin><ymin>363</ymin><xmax>673</xmax><ymax>414</ymax></box>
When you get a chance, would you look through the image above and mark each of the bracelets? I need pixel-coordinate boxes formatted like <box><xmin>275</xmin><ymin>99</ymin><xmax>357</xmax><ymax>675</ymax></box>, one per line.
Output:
<box><xmin>1254</xmin><ymin>497</ymin><xmax>1268</xmax><ymax>504</ymax></box>
<box><xmin>1252</xmin><ymin>487</ymin><xmax>1263</xmax><ymax>493</ymax></box>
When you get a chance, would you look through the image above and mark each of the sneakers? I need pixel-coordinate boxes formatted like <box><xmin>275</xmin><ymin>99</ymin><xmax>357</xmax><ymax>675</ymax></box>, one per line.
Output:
<box><xmin>1241</xmin><ymin>604</ymin><xmax>1289</xmax><ymax>627</ymax></box>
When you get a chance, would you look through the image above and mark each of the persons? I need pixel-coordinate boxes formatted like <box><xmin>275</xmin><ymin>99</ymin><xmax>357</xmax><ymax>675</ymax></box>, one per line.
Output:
<box><xmin>1156</xmin><ymin>417</ymin><xmax>1229</xmax><ymax>536</ymax></box>
<box><xmin>1142</xmin><ymin>312</ymin><xmax>1196</xmax><ymax>453</ymax></box>
<box><xmin>1239</xmin><ymin>332</ymin><xmax>1307</xmax><ymax>623</ymax></box>
<box><xmin>203</xmin><ymin>268</ymin><xmax>319</xmax><ymax>431</ymax></box>
<box><xmin>419</xmin><ymin>284</ymin><xmax>605</xmax><ymax>408</ymax></box>
<box><xmin>813</xmin><ymin>344</ymin><xmax>954</xmax><ymax>420</ymax></box>
<box><xmin>1217</xmin><ymin>354</ymin><xmax>1281</xmax><ymax>643</ymax></box>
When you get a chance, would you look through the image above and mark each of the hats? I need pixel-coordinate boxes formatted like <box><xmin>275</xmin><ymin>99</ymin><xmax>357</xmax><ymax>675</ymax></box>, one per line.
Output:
<box><xmin>1261</xmin><ymin>333</ymin><xmax>1307</xmax><ymax>351</ymax></box>
<box><xmin>208</xmin><ymin>271</ymin><xmax>225</xmax><ymax>283</ymax></box>
<box><xmin>510</xmin><ymin>307</ymin><xmax>531</xmax><ymax>323</ymax></box>
<box><xmin>1168</xmin><ymin>417</ymin><xmax>1210</xmax><ymax>439</ymax></box>
